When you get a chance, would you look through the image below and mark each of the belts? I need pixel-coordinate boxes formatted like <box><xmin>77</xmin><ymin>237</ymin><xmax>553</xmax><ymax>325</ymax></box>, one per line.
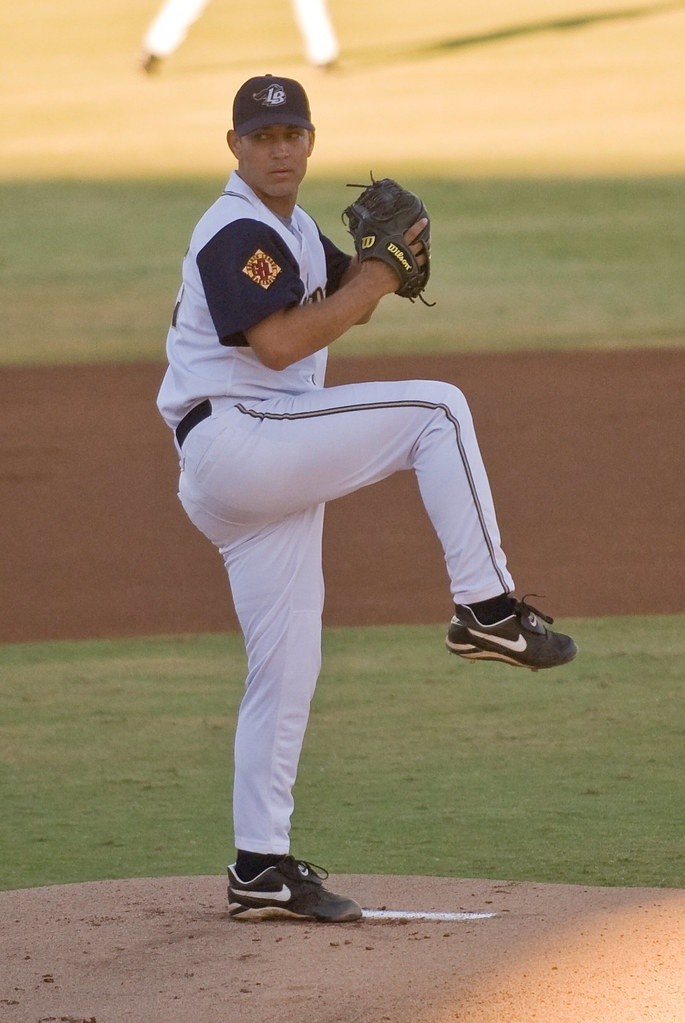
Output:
<box><xmin>174</xmin><ymin>398</ymin><xmax>212</xmax><ymax>449</ymax></box>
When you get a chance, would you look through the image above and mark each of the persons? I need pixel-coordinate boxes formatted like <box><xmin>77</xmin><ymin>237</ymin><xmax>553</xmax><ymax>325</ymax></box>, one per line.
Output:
<box><xmin>139</xmin><ymin>0</ymin><xmax>347</xmax><ymax>77</ymax></box>
<box><xmin>155</xmin><ymin>74</ymin><xmax>579</xmax><ymax>925</ymax></box>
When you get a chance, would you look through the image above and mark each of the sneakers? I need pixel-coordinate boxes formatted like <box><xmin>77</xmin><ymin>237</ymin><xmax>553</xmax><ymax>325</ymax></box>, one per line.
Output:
<box><xmin>444</xmin><ymin>602</ymin><xmax>580</xmax><ymax>671</ymax></box>
<box><xmin>226</xmin><ymin>856</ymin><xmax>362</xmax><ymax>924</ymax></box>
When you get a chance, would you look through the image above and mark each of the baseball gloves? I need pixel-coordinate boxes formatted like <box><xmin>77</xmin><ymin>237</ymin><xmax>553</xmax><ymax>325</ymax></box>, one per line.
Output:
<box><xmin>340</xmin><ymin>171</ymin><xmax>438</xmax><ymax>307</ymax></box>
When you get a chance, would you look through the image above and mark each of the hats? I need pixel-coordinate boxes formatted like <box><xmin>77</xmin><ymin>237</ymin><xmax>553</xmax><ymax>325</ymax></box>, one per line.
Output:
<box><xmin>232</xmin><ymin>74</ymin><xmax>313</xmax><ymax>137</ymax></box>
